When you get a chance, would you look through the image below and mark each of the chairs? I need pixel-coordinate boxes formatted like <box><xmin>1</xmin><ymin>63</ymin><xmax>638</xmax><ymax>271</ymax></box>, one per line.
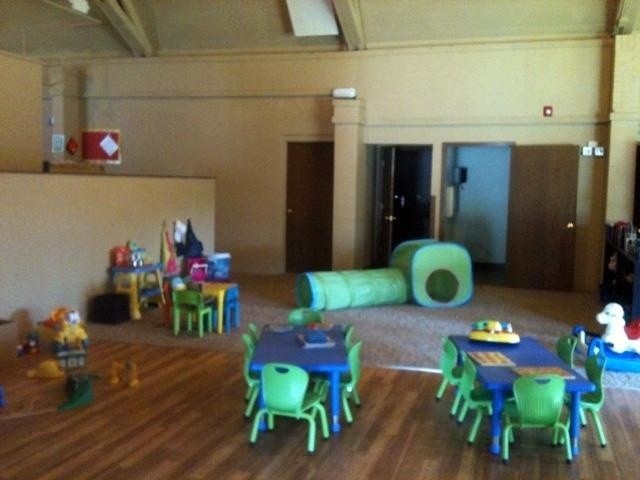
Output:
<box><xmin>436</xmin><ymin>320</ymin><xmax>607</xmax><ymax>464</ymax></box>
<box><xmin>242</xmin><ymin>307</ymin><xmax>362</xmax><ymax>455</ymax></box>
<box><xmin>224</xmin><ymin>287</ymin><xmax>240</xmax><ymax>334</ymax></box>
<box><xmin>170</xmin><ymin>289</ymin><xmax>213</xmax><ymax>337</ymax></box>
<box><xmin>163</xmin><ymin>281</ymin><xmax>195</xmax><ymax>330</ymax></box>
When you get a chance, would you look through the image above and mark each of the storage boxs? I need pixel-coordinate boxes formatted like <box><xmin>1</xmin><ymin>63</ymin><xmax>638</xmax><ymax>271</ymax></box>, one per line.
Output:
<box><xmin>206</xmin><ymin>252</ymin><xmax>232</xmax><ymax>280</ymax></box>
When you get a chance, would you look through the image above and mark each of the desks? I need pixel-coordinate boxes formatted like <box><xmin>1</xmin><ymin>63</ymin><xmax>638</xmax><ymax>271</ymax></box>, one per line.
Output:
<box><xmin>199</xmin><ymin>282</ymin><xmax>237</xmax><ymax>333</ymax></box>
<box><xmin>112</xmin><ymin>263</ymin><xmax>166</xmax><ymax>321</ymax></box>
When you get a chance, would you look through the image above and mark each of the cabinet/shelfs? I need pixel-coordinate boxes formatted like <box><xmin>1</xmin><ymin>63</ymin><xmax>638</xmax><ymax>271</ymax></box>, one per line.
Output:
<box><xmin>602</xmin><ymin>223</ymin><xmax>639</xmax><ymax>326</ymax></box>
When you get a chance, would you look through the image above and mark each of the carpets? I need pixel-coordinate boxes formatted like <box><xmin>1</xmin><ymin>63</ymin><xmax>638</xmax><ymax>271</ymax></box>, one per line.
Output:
<box><xmin>82</xmin><ymin>272</ymin><xmax>640</xmax><ymax>391</ymax></box>
